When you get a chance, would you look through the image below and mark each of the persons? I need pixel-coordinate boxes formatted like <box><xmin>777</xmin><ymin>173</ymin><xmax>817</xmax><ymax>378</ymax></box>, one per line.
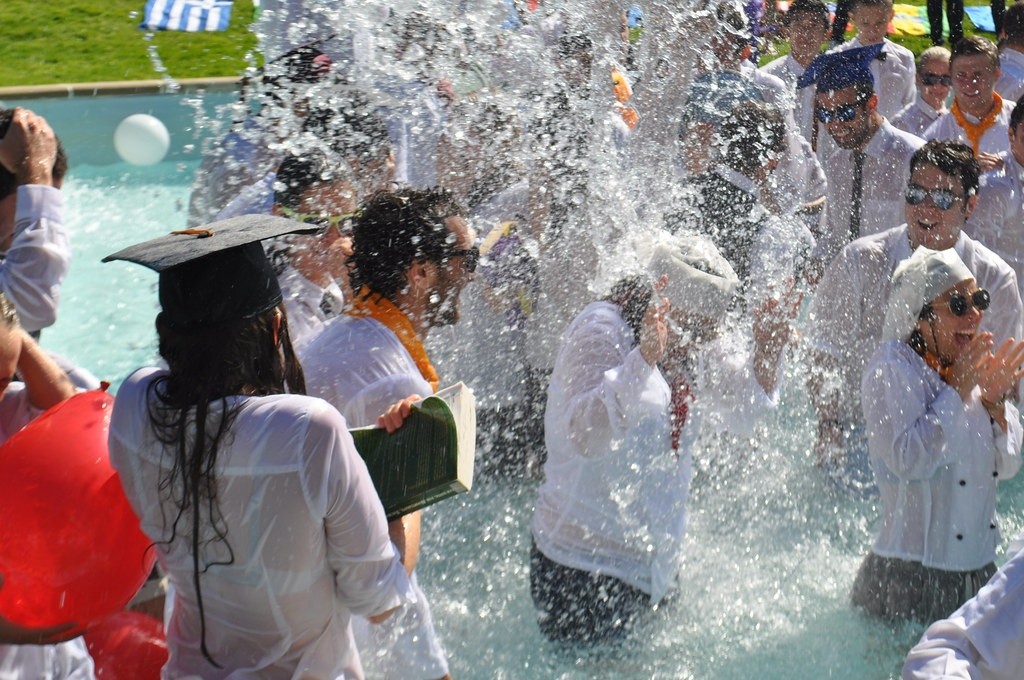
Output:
<box><xmin>0</xmin><ymin>287</ymin><xmax>94</xmax><ymax>680</ymax></box>
<box><xmin>190</xmin><ymin>0</ymin><xmax>1024</xmax><ymax>680</ymax></box>
<box><xmin>109</xmin><ymin>214</ymin><xmax>425</xmax><ymax>679</ymax></box>
<box><xmin>0</xmin><ymin>106</ymin><xmax>69</xmax><ymax>343</ymax></box>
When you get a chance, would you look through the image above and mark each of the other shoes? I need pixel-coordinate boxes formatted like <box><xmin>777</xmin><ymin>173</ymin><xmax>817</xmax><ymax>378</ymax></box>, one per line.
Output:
<box><xmin>931</xmin><ymin>34</ymin><xmax>943</xmax><ymax>46</ymax></box>
<box><xmin>948</xmin><ymin>34</ymin><xmax>964</xmax><ymax>43</ymax></box>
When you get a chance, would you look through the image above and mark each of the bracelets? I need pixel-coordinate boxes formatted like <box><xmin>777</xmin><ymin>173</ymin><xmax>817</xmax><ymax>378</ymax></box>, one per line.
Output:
<box><xmin>981</xmin><ymin>394</ymin><xmax>1005</xmax><ymax>408</ymax></box>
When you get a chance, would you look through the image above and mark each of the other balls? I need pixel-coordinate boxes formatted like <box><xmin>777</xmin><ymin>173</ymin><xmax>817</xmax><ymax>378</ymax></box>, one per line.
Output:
<box><xmin>114</xmin><ymin>113</ymin><xmax>172</xmax><ymax>166</ymax></box>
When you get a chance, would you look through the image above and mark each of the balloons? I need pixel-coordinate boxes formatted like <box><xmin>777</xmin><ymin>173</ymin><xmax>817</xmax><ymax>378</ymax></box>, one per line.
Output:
<box><xmin>84</xmin><ymin>612</ymin><xmax>169</xmax><ymax>680</ymax></box>
<box><xmin>114</xmin><ymin>114</ymin><xmax>170</xmax><ymax>166</ymax></box>
<box><xmin>0</xmin><ymin>382</ymin><xmax>158</xmax><ymax>636</ymax></box>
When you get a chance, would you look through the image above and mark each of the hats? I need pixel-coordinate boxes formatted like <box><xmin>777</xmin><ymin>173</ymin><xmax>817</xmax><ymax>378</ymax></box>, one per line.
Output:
<box><xmin>796</xmin><ymin>41</ymin><xmax>887</xmax><ymax>92</ymax></box>
<box><xmin>101</xmin><ymin>213</ymin><xmax>322</xmax><ymax>319</ymax></box>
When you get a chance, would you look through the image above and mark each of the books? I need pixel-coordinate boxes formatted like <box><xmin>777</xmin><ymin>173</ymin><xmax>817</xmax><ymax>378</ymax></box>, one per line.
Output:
<box><xmin>348</xmin><ymin>382</ymin><xmax>477</xmax><ymax>522</ymax></box>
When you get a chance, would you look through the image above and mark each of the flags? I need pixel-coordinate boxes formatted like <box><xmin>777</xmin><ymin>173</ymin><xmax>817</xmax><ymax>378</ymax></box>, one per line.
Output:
<box><xmin>139</xmin><ymin>0</ymin><xmax>233</xmax><ymax>32</ymax></box>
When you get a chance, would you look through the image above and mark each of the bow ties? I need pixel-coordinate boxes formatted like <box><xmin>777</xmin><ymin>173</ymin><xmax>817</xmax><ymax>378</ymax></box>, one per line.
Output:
<box><xmin>874</xmin><ymin>52</ymin><xmax>886</xmax><ymax>61</ymax></box>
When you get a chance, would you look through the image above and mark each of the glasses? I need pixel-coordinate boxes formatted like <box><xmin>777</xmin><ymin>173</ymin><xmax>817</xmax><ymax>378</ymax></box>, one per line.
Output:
<box><xmin>817</xmin><ymin>91</ymin><xmax>875</xmax><ymax>125</ymax></box>
<box><xmin>924</xmin><ymin>288</ymin><xmax>991</xmax><ymax>318</ymax></box>
<box><xmin>278</xmin><ymin>205</ymin><xmax>362</xmax><ymax>238</ymax></box>
<box><xmin>445</xmin><ymin>246</ymin><xmax>480</xmax><ymax>273</ymax></box>
<box><xmin>905</xmin><ymin>183</ymin><xmax>969</xmax><ymax>211</ymax></box>
<box><xmin>918</xmin><ymin>71</ymin><xmax>951</xmax><ymax>87</ymax></box>
